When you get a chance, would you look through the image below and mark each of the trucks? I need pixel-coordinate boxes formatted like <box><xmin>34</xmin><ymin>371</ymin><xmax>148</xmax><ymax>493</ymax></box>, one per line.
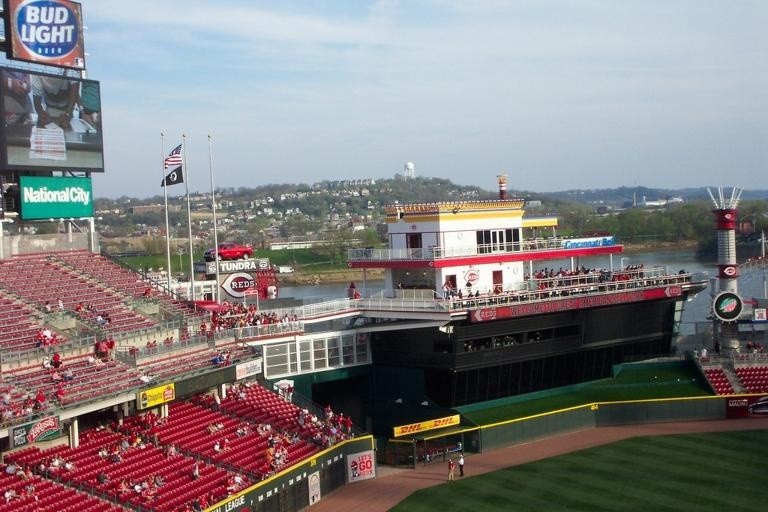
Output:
<box><xmin>276</xmin><ymin>266</ymin><xmax>295</xmax><ymax>274</ymax></box>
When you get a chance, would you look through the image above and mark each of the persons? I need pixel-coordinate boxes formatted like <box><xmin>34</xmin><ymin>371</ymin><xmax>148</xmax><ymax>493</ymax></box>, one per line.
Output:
<box><xmin>1</xmin><ymin>288</ymin><xmax>354</xmax><ymax>512</ymax></box>
<box><xmin>427</xmin><ymin>448</ymin><xmax>433</xmax><ymax>464</ymax></box>
<box><xmin>701</xmin><ymin>347</ymin><xmax>707</xmax><ymax>359</ymax></box>
<box><xmin>715</xmin><ymin>341</ymin><xmax>720</xmax><ymax>353</ymax></box>
<box><xmin>442</xmin><ymin>264</ymin><xmax>692</xmax><ymax>308</ymax></box>
<box><xmin>1</xmin><ymin>70</ymin><xmax>100</xmax><ymax>129</ymax></box>
<box><xmin>747</xmin><ymin>340</ymin><xmax>765</xmax><ymax>354</ymax></box>
<box><xmin>447</xmin><ymin>460</ymin><xmax>455</xmax><ymax>481</ymax></box>
<box><xmin>458</xmin><ymin>454</ymin><xmax>464</xmax><ymax>476</ymax></box>
<box><xmin>693</xmin><ymin>348</ymin><xmax>699</xmax><ymax>359</ymax></box>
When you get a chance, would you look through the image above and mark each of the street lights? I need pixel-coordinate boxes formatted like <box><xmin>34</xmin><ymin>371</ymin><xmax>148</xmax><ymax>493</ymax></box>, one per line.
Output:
<box><xmin>176</xmin><ymin>247</ymin><xmax>186</xmax><ymax>272</ymax></box>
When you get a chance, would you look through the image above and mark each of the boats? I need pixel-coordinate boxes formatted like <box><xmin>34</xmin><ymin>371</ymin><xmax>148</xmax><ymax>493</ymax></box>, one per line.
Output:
<box><xmin>137</xmin><ymin>265</ymin><xmax>179</xmax><ymax>285</ymax></box>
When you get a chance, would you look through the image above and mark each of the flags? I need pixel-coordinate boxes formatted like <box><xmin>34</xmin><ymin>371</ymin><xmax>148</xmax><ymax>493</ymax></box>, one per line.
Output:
<box><xmin>165</xmin><ymin>143</ymin><xmax>183</xmax><ymax>169</ymax></box>
<box><xmin>161</xmin><ymin>166</ymin><xmax>184</xmax><ymax>187</ymax></box>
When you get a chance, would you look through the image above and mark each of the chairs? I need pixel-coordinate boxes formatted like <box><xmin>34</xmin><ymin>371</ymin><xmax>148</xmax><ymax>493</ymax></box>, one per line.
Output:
<box><xmin>704</xmin><ymin>366</ymin><xmax>768</xmax><ymax>395</ymax></box>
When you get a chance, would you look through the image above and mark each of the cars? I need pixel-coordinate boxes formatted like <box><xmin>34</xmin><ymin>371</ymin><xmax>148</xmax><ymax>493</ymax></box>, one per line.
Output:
<box><xmin>203</xmin><ymin>242</ymin><xmax>255</xmax><ymax>262</ymax></box>
<box><xmin>748</xmin><ymin>395</ymin><xmax>768</xmax><ymax>416</ymax></box>
